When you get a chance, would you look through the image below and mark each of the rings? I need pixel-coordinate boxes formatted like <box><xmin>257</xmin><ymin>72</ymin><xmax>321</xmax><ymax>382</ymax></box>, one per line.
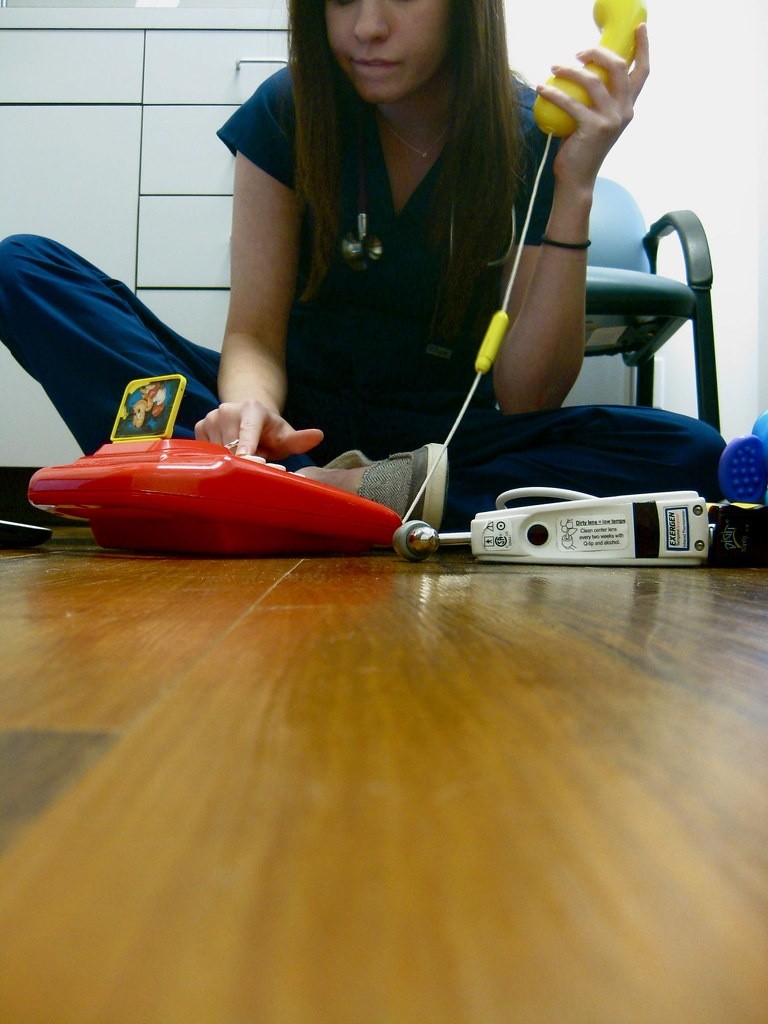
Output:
<box><xmin>224</xmin><ymin>439</ymin><xmax>239</xmax><ymax>451</ymax></box>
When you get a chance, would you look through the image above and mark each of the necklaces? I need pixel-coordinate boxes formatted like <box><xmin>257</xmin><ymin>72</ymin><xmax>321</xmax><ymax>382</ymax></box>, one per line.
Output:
<box><xmin>382</xmin><ymin>117</ymin><xmax>449</xmax><ymax>158</ymax></box>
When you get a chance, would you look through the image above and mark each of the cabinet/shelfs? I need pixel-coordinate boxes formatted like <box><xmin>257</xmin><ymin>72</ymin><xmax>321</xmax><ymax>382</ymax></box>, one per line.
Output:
<box><xmin>0</xmin><ymin>6</ymin><xmax>290</xmax><ymax>467</ymax></box>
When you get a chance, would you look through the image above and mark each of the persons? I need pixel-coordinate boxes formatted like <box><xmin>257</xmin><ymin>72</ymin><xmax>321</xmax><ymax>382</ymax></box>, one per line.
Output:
<box><xmin>0</xmin><ymin>0</ymin><xmax>728</xmax><ymax>533</ymax></box>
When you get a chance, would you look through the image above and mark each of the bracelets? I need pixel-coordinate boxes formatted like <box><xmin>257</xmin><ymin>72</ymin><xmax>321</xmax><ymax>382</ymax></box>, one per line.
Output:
<box><xmin>541</xmin><ymin>234</ymin><xmax>592</xmax><ymax>250</ymax></box>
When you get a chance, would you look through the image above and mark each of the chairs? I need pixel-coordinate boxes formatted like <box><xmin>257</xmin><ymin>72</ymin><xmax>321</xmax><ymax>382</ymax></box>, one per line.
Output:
<box><xmin>585</xmin><ymin>177</ymin><xmax>720</xmax><ymax>435</ymax></box>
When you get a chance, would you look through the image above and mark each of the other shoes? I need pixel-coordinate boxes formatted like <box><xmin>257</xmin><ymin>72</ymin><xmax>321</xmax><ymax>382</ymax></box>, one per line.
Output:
<box><xmin>322</xmin><ymin>442</ymin><xmax>447</xmax><ymax>533</ymax></box>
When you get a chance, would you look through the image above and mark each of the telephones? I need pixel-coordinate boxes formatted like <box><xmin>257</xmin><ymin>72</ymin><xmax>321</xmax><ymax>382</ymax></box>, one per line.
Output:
<box><xmin>533</xmin><ymin>0</ymin><xmax>648</xmax><ymax>138</ymax></box>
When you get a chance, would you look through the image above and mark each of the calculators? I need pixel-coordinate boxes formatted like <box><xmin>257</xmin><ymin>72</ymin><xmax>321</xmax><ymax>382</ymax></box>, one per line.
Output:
<box><xmin>0</xmin><ymin>521</ymin><xmax>53</xmax><ymax>550</ymax></box>
